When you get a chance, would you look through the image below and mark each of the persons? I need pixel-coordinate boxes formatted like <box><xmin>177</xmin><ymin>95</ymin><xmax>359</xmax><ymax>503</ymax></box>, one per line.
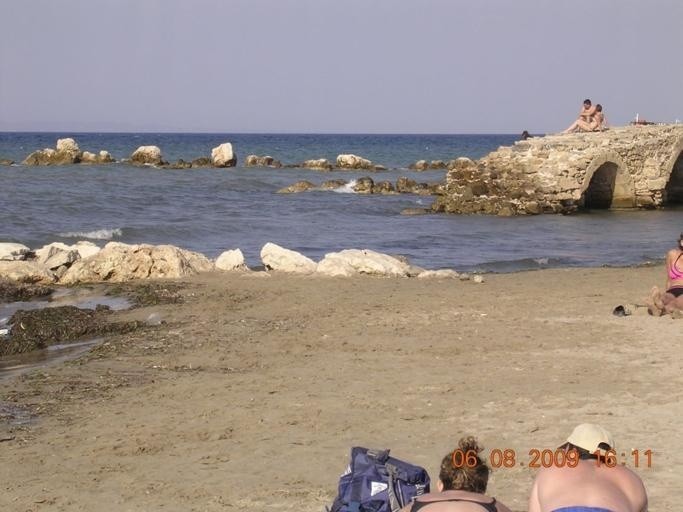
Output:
<box><xmin>651</xmin><ymin>230</ymin><xmax>683</xmax><ymax>316</ymax></box>
<box><xmin>528</xmin><ymin>423</ymin><xmax>648</xmax><ymax>512</ymax></box>
<box><xmin>575</xmin><ymin>98</ymin><xmax>597</xmax><ymax>122</ymax></box>
<box><xmin>522</xmin><ymin>131</ymin><xmax>530</xmax><ymax>138</ymax></box>
<box><xmin>396</xmin><ymin>435</ymin><xmax>511</xmax><ymax>512</ymax></box>
<box><xmin>561</xmin><ymin>104</ymin><xmax>608</xmax><ymax>134</ymax></box>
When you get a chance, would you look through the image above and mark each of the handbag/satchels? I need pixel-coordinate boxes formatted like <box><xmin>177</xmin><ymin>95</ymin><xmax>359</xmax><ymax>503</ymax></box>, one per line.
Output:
<box><xmin>330</xmin><ymin>448</ymin><xmax>431</xmax><ymax>512</ymax></box>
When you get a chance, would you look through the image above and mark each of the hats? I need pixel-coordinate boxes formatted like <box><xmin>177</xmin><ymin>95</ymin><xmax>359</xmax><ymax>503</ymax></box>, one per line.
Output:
<box><xmin>560</xmin><ymin>423</ymin><xmax>615</xmax><ymax>457</ymax></box>
